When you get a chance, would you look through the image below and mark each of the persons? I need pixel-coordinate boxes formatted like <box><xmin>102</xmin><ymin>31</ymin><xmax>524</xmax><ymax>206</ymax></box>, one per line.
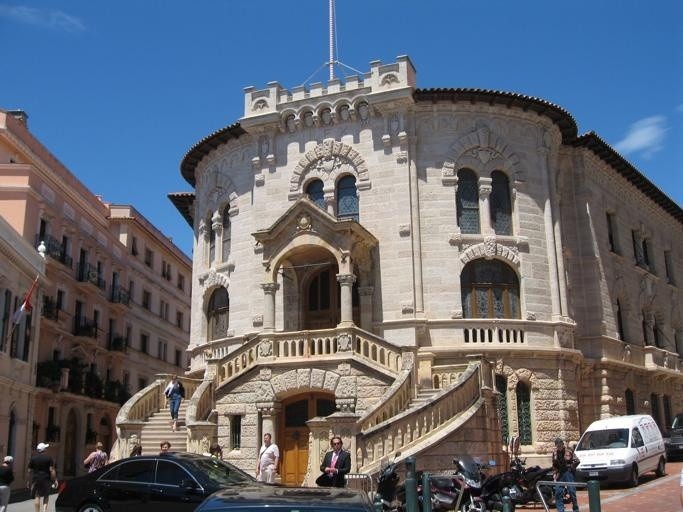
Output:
<box><xmin>550</xmin><ymin>437</ymin><xmax>581</xmax><ymax>512</ymax></box>
<box><xmin>318</xmin><ymin>436</ymin><xmax>351</xmax><ymax>488</ymax></box>
<box><xmin>254</xmin><ymin>433</ymin><xmax>280</xmax><ymax>484</ymax></box>
<box><xmin>200</xmin><ymin>442</ymin><xmax>222</xmax><ymax>482</ymax></box>
<box><xmin>129</xmin><ymin>443</ymin><xmax>142</xmax><ymax>458</ymax></box>
<box><xmin>164</xmin><ymin>374</ymin><xmax>184</xmax><ymax>432</ymax></box>
<box><xmin>83</xmin><ymin>441</ymin><xmax>109</xmax><ymax>475</ymax></box>
<box><xmin>160</xmin><ymin>441</ymin><xmax>171</xmax><ymax>454</ymax></box>
<box><xmin>0</xmin><ymin>456</ymin><xmax>14</xmax><ymax>512</ymax></box>
<box><xmin>24</xmin><ymin>443</ymin><xmax>59</xmax><ymax>512</ymax></box>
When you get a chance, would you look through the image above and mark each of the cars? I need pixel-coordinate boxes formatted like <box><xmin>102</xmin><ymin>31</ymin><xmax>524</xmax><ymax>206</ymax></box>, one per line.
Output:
<box><xmin>54</xmin><ymin>453</ymin><xmax>259</xmax><ymax>511</ymax></box>
<box><xmin>661</xmin><ymin>411</ymin><xmax>682</xmax><ymax>459</ymax></box>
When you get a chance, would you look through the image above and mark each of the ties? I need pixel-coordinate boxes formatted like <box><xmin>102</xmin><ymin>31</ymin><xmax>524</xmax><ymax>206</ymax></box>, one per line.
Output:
<box><xmin>329</xmin><ymin>454</ymin><xmax>337</xmax><ymax>475</ymax></box>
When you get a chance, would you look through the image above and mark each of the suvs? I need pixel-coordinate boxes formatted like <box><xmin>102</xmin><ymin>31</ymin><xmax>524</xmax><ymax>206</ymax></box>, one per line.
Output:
<box><xmin>197</xmin><ymin>482</ymin><xmax>378</xmax><ymax>511</ymax></box>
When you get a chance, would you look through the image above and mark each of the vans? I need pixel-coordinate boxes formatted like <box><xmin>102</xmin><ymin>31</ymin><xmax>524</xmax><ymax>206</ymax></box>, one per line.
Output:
<box><xmin>571</xmin><ymin>414</ymin><xmax>665</xmax><ymax>487</ymax></box>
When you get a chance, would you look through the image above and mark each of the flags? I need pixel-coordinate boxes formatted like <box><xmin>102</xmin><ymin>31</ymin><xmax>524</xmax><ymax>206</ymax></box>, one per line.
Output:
<box><xmin>10</xmin><ymin>278</ymin><xmax>38</xmax><ymax>325</ymax></box>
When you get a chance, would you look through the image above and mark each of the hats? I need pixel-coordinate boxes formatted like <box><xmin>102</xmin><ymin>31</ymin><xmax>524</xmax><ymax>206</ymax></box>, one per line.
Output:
<box><xmin>37</xmin><ymin>443</ymin><xmax>49</xmax><ymax>452</ymax></box>
<box><xmin>555</xmin><ymin>438</ymin><xmax>563</xmax><ymax>445</ymax></box>
<box><xmin>3</xmin><ymin>456</ymin><xmax>13</xmax><ymax>463</ymax></box>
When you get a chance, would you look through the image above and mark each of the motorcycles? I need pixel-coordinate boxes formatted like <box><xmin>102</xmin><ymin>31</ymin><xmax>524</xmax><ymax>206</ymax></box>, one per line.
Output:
<box><xmin>371</xmin><ymin>434</ymin><xmax>576</xmax><ymax>512</ymax></box>
